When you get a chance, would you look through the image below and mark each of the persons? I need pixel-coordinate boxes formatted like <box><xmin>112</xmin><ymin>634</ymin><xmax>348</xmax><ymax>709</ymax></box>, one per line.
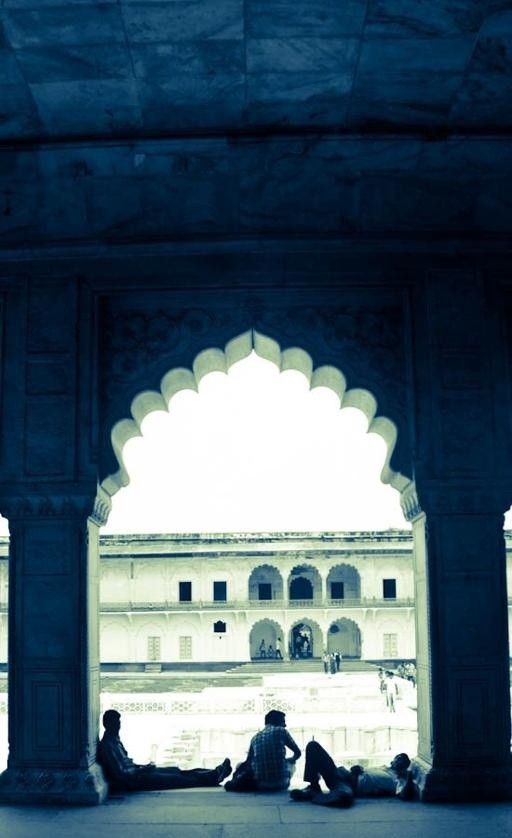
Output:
<box><xmin>296</xmin><ymin>632</ymin><xmax>309</xmax><ymax>656</ymax></box>
<box><xmin>100</xmin><ymin>709</ymin><xmax>232</xmax><ymax>791</ymax></box>
<box><xmin>243</xmin><ymin>709</ymin><xmax>302</xmax><ymax>791</ymax></box>
<box><xmin>321</xmin><ymin>648</ymin><xmax>341</xmax><ymax>675</ymax></box>
<box><xmin>398</xmin><ymin>660</ymin><xmax>416</xmax><ymax>688</ymax></box>
<box><xmin>377</xmin><ymin>667</ymin><xmax>399</xmax><ymax>715</ymax></box>
<box><xmin>260</xmin><ymin>637</ymin><xmax>283</xmax><ymax>660</ymax></box>
<box><xmin>289</xmin><ymin>741</ymin><xmax>415</xmax><ymax>808</ymax></box>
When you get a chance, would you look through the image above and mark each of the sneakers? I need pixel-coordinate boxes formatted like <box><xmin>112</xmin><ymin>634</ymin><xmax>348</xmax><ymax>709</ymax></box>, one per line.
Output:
<box><xmin>291</xmin><ymin>785</ymin><xmax>321</xmax><ymax>800</ymax></box>
<box><xmin>216</xmin><ymin>759</ymin><xmax>232</xmax><ymax>782</ymax></box>
